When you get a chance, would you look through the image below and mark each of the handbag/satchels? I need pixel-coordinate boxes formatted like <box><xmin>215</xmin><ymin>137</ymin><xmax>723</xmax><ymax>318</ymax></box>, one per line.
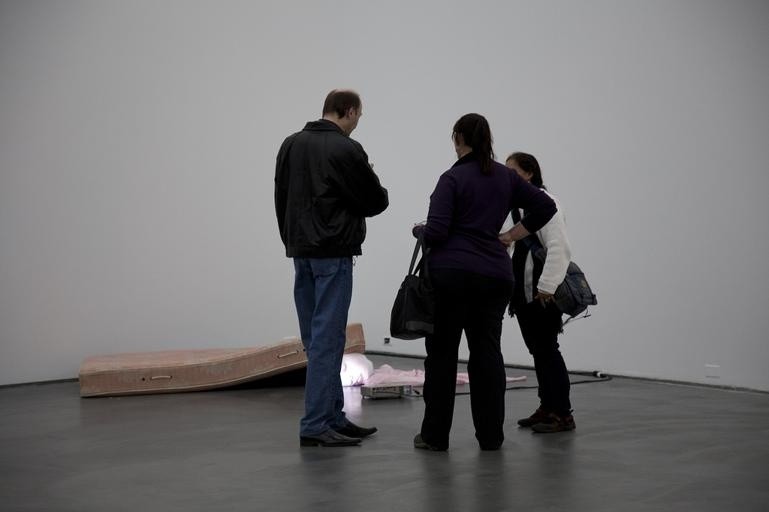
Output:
<box><xmin>552</xmin><ymin>261</ymin><xmax>598</xmax><ymax>318</ymax></box>
<box><xmin>390</xmin><ymin>272</ymin><xmax>437</xmax><ymax>341</ymax></box>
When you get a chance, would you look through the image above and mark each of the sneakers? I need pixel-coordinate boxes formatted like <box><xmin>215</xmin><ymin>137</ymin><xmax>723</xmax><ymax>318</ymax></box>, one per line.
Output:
<box><xmin>530</xmin><ymin>409</ymin><xmax>576</xmax><ymax>433</ymax></box>
<box><xmin>478</xmin><ymin>434</ymin><xmax>504</xmax><ymax>451</ymax></box>
<box><xmin>517</xmin><ymin>407</ymin><xmax>551</xmax><ymax>427</ymax></box>
<box><xmin>413</xmin><ymin>432</ymin><xmax>449</xmax><ymax>451</ymax></box>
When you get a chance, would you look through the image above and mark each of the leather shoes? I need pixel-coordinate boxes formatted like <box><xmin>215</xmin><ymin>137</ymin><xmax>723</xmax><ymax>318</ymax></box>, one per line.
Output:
<box><xmin>299</xmin><ymin>424</ymin><xmax>363</xmax><ymax>447</ymax></box>
<box><xmin>330</xmin><ymin>417</ymin><xmax>378</xmax><ymax>439</ymax></box>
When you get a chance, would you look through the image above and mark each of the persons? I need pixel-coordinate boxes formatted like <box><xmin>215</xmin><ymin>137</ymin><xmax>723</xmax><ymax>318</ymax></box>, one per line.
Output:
<box><xmin>506</xmin><ymin>150</ymin><xmax>576</xmax><ymax>434</ymax></box>
<box><xmin>273</xmin><ymin>89</ymin><xmax>379</xmax><ymax>448</ymax></box>
<box><xmin>411</xmin><ymin>110</ymin><xmax>558</xmax><ymax>450</ymax></box>
